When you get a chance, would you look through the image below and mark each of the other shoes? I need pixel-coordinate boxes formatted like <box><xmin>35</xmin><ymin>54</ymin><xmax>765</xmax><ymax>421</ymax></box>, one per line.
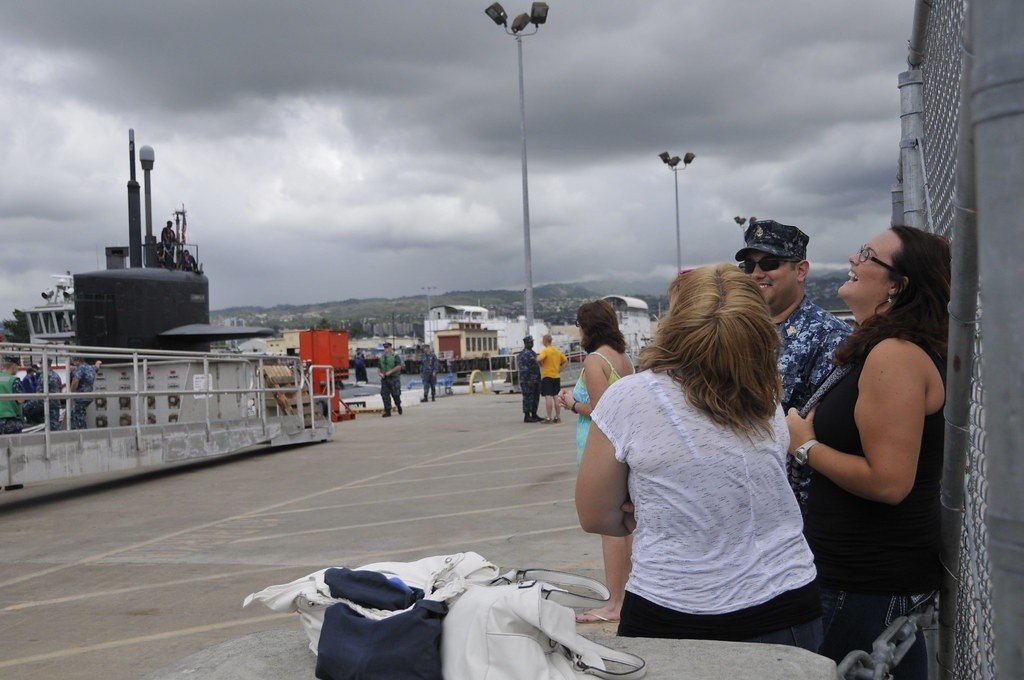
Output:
<box><xmin>524</xmin><ymin>416</ymin><xmax>539</xmax><ymax>422</ymax></box>
<box><xmin>398</xmin><ymin>406</ymin><xmax>402</xmax><ymax>414</ymax></box>
<box><xmin>541</xmin><ymin>418</ymin><xmax>553</xmax><ymax>423</ymax></box>
<box><xmin>383</xmin><ymin>413</ymin><xmax>391</xmax><ymax>417</ymax></box>
<box><xmin>432</xmin><ymin>398</ymin><xmax>434</xmax><ymax>401</ymax></box>
<box><xmin>552</xmin><ymin>417</ymin><xmax>559</xmax><ymax>422</ymax></box>
<box><xmin>421</xmin><ymin>398</ymin><xmax>427</xmax><ymax>401</ymax></box>
<box><xmin>532</xmin><ymin>415</ymin><xmax>544</xmax><ymax>420</ymax></box>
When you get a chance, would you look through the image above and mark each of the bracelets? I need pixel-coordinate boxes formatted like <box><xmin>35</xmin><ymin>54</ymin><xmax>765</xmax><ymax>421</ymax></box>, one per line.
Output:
<box><xmin>571</xmin><ymin>401</ymin><xmax>578</xmax><ymax>416</ymax></box>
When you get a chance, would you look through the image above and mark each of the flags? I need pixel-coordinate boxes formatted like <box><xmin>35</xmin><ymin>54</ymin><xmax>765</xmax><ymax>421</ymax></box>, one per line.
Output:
<box><xmin>181</xmin><ymin>214</ymin><xmax>187</xmax><ymax>246</ymax></box>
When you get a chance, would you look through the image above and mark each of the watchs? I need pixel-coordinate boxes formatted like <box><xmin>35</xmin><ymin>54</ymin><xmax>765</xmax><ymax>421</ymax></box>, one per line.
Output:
<box><xmin>794</xmin><ymin>439</ymin><xmax>819</xmax><ymax>465</ymax></box>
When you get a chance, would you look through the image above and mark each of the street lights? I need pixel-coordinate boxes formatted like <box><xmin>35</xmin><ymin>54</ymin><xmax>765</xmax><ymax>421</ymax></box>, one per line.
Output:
<box><xmin>483</xmin><ymin>0</ymin><xmax>550</xmax><ymax>338</ymax></box>
<box><xmin>659</xmin><ymin>151</ymin><xmax>696</xmax><ymax>269</ymax></box>
<box><xmin>421</xmin><ymin>284</ymin><xmax>440</xmax><ymax>353</ymax></box>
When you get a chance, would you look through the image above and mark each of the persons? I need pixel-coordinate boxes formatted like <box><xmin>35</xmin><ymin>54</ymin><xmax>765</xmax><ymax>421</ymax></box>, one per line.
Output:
<box><xmin>70</xmin><ymin>357</ymin><xmax>102</xmax><ymax>430</ymax></box>
<box><xmin>535</xmin><ymin>335</ymin><xmax>568</xmax><ymax>424</ymax></box>
<box><xmin>162</xmin><ymin>221</ymin><xmax>180</xmax><ymax>270</ymax></box>
<box><xmin>378</xmin><ymin>342</ymin><xmax>402</xmax><ymax>418</ymax></box>
<box><xmin>22</xmin><ymin>358</ymin><xmax>62</xmax><ymax>431</ymax></box>
<box><xmin>352</xmin><ymin>348</ymin><xmax>369</xmax><ymax>385</ymax></box>
<box><xmin>557</xmin><ymin>299</ymin><xmax>636</xmax><ymax>624</ymax></box>
<box><xmin>180</xmin><ymin>244</ymin><xmax>204</xmax><ymax>274</ymax></box>
<box><xmin>0</xmin><ymin>356</ymin><xmax>26</xmax><ymax>434</ymax></box>
<box><xmin>736</xmin><ymin>219</ymin><xmax>854</xmax><ymax>654</ymax></box>
<box><xmin>419</xmin><ymin>345</ymin><xmax>438</xmax><ymax>402</ymax></box>
<box><xmin>518</xmin><ymin>335</ymin><xmax>545</xmax><ymax>422</ymax></box>
<box><xmin>22</xmin><ymin>369</ymin><xmax>38</xmax><ymax>393</ymax></box>
<box><xmin>784</xmin><ymin>225</ymin><xmax>952</xmax><ymax>680</ymax></box>
<box><xmin>61</xmin><ymin>324</ymin><xmax>73</xmax><ymax>332</ymax></box>
<box><xmin>576</xmin><ymin>261</ymin><xmax>825</xmax><ymax>652</ymax></box>
<box><xmin>31</xmin><ymin>364</ymin><xmax>40</xmax><ymax>380</ymax></box>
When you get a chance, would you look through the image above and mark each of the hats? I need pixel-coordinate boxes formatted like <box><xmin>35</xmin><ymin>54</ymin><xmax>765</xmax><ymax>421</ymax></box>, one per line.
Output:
<box><xmin>72</xmin><ymin>355</ymin><xmax>81</xmax><ymax>360</ymax></box>
<box><xmin>383</xmin><ymin>342</ymin><xmax>392</xmax><ymax>346</ymax></box>
<box><xmin>735</xmin><ymin>220</ymin><xmax>809</xmax><ymax>259</ymax></box>
<box><xmin>5</xmin><ymin>356</ymin><xmax>22</xmax><ymax>367</ymax></box>
<box><xmin>523</xmin><ymin>336</ymin><xmax>535</xmax><ymax>343</ymax></box>
<box><xmin>39</xmin><ymin>357</ymin><xmax>53</xmax><ymax>366</ymax></box>
<box><xmin>422</xmin><ymin>345</ymin><xmax>430</xmax><ymax>348</ymax></box>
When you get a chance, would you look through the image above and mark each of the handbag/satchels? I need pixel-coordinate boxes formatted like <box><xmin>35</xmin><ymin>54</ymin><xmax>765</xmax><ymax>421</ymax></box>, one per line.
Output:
<box><xmin>323</xmin><ymin>568</ymin><xmax>425</xmax><ymax>611</ymax></box>
<box><xmin>354</xmin><ymin>359</ymin><xmax>361</xmax><ymax>366</ymax></box>
<box><xmin>440</xmin><ymin>568</ymin><xmax>647</xmax><ymax>680</ymax></box>
<box><xmin>315</xmin><ymin>600</ymin><xmax>449</xmax><ymax>680</ymax></box>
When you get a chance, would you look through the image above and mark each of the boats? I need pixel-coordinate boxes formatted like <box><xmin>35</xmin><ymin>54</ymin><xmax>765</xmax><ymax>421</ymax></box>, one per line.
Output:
<box><xmin>0</xmin><ymin>270</ymin><xmax>76</xmax><ymax>432</ymax></box>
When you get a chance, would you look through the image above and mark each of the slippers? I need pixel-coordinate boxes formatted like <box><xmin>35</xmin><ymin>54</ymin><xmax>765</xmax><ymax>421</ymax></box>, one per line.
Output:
<box><xmin>575</xmin><ymin>609</ymin><xmax>621</xmax><ymax>622</ymax></box>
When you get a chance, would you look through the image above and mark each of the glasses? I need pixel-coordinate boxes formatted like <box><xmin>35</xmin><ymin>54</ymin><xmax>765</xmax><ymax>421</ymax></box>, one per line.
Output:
<box><xmin>856</xmin><ymin>243</ymin><xmax>904</xmax><ymax>276</ymax></box>
<box><xmin>737</xmin><ymin>256</ymin><xmax>803</xmax><ymax>274</ymax></box>
<box><xmin>678</xmin><ymin>269</ymin><xmax>694</xmax><ymax>289</ymax></box>
<box><xmin>575</xmin><ymin>319</ymin><xmax>585</xmax><ymax>327</ymax></box>
<box><xmin>384</xmin><ymin>346</ymin><xmax>390</xmax><ymax>348</ymax></box>
